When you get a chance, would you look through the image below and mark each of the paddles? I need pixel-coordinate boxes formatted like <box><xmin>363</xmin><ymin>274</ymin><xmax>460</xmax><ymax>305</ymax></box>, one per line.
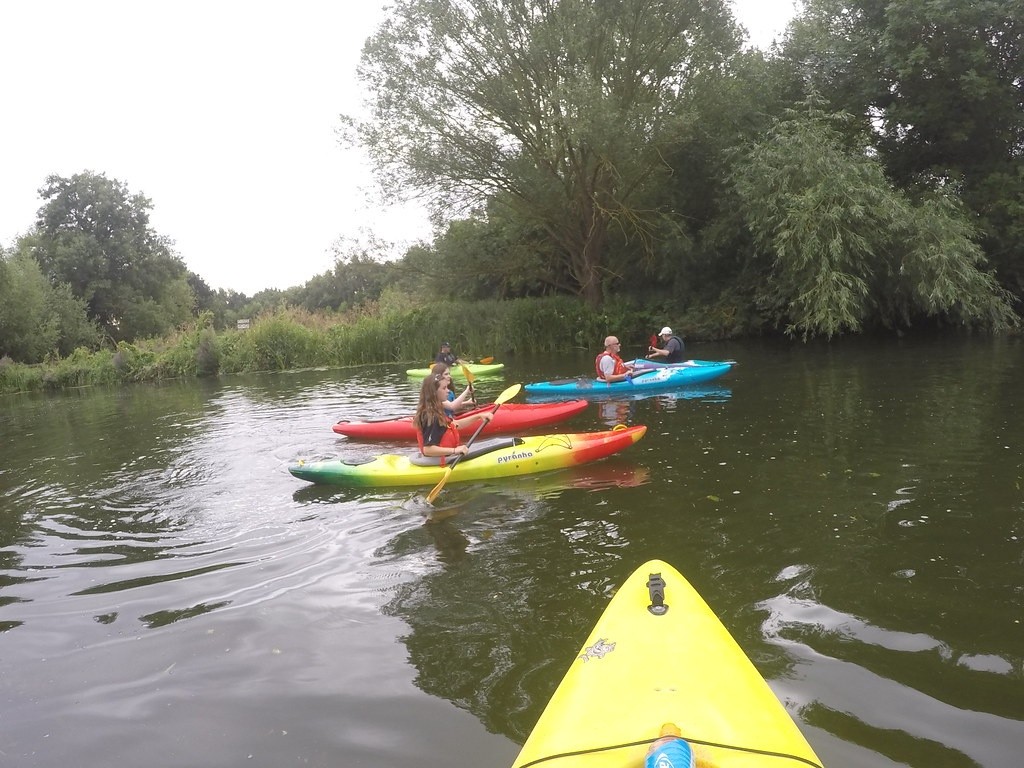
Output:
<box><xmin>647</xmin><ymin>335</ymin><xmax>658</xmax><ymax>359</ymax></box>
<box><xmin>624</xmin><ymin>356</ymin><xmax>639</xmax><ymax>387</ymax></box>
<box><xmin>426</xmin><ymin>382</ymin><xmax>522</xmax><ymax>505</ymax></box>
<box><xmin>460</xmin><ymin>364</ymin><xmax>478</xmax><ymax>408</ymax></box>
<box><xmin>429</xmin><ymin>356</ymin><xmax>494</xmax><ymax>370</ymax></box>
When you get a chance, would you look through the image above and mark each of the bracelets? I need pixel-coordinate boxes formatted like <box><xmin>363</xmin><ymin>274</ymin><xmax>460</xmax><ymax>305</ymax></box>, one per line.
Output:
<box><xmin>454</xmin><ymin>448</ymin><xmax>455</xmax><ymax>454</ymax></box>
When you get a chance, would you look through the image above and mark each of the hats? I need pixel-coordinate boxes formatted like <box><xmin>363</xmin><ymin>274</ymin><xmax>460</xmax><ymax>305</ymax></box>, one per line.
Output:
<box><xmin>658</xmin><ymin>327</ymin><xmax>672</xmax><ymax>336</ymax></box>
<box><xmin>441</xmin><ymin>341</ymin><xmax>451</xmax><ymax>347</ymax></box>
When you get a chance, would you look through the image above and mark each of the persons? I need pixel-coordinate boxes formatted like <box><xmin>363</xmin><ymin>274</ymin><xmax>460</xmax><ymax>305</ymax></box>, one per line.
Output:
<box><xmin>430</xmin><ymin>364</ymin><xmax>477</xmax><ymax>417</ymax></box>
<box><xmin>596</xmin><ymin>336</ymin><xmax>636</xmax><ymax>383</ymax></box>
<box><xmin>437</xmin><ymin>341</ymin><xmax>461</xmax><ymax>367</ymax></box>
<box><xmin>645</xmin><ymin>326</ymin><xmax>686</xmax><ymax>364</ymax></box>
<box><xmin>416</xmin><ymin>374</ymin><xmax>495</xmax><ymax>456</ymax></box>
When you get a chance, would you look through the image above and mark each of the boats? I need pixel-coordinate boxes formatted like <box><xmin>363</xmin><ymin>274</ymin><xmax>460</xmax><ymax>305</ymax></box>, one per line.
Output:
<box><xmin>524</xmin><ymin>357</ymin><xmax>737</xmax><ymax>396</ymax></box>
<box><xmin>333</xmin><ymin>400</ymin><xmax>589</xmax><ymax>440</ymax></box>
<box><xmin>406</xmin><ymin>362</ymin><xmax>505</xmax><ymax>378</ymax></box>
<box><xmin>288</xmin><ymin>423</ymin><xmax>648</xmax><ymax>486</ymax></box>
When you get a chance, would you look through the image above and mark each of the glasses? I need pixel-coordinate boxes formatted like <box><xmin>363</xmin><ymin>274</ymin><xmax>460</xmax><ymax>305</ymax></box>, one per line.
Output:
<box><xmin>608</xmin><ymin>341</ymin><xmax>620</xmax><ymax>345</ymax></box>
